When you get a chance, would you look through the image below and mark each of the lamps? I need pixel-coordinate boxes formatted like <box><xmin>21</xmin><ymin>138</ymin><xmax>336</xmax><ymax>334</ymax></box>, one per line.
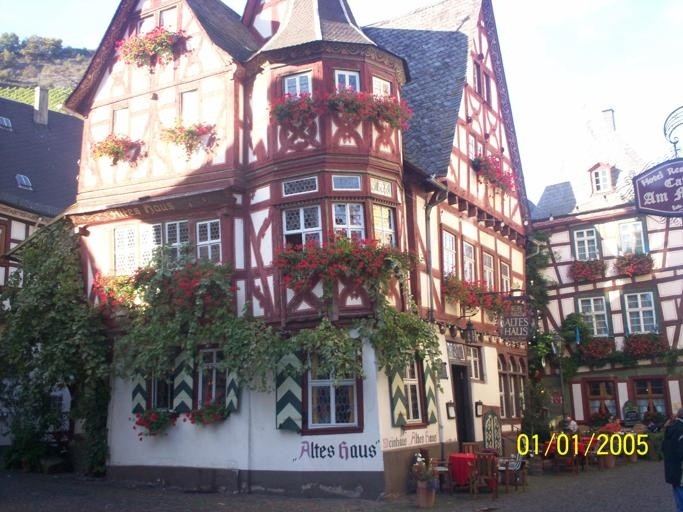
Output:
<box><xmin>448</xmin><ymin>309</ymin><xmax>481</xmax><ymax>344</ymax></box>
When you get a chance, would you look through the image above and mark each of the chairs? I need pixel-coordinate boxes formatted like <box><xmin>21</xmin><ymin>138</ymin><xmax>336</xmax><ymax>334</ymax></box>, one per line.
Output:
<box><xmin>413</xmin><ymin>418</ymin><xmax>643</xmax><ymax>508</ymax></box>
